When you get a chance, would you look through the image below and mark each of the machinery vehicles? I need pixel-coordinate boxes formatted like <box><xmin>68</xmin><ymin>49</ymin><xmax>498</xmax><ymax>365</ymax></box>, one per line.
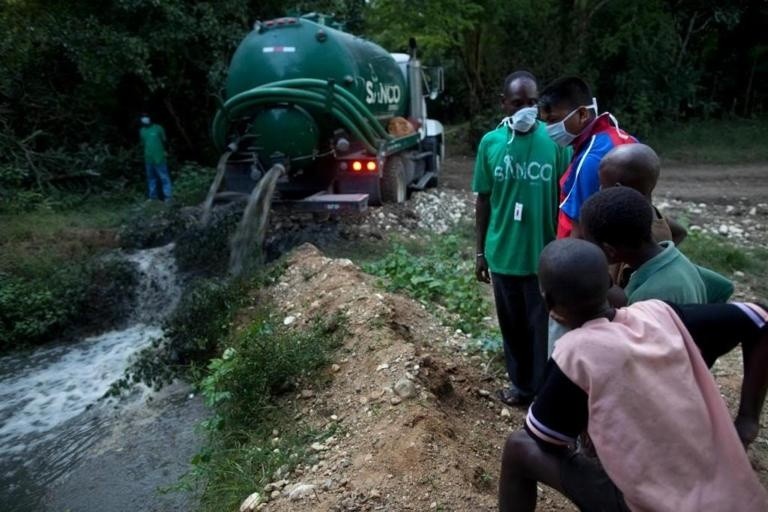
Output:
<box><xmin>211</xmin><ymin>11</ymin><xmax>444</xmax><ymax>223</ymax></box>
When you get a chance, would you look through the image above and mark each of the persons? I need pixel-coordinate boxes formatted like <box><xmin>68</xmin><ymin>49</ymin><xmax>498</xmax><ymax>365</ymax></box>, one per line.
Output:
<box><xmin>139</xmin><ymin>109</ymin><xmax>173</xmax><ymax>201</ymax></box>
<box><xmin>496</xmin><ymin>239</ymin><xmax>767</xmax><ymax>511</ymax></box>
<box><xmin>470</xmin><ymin>70</ymin><xmax>573</xmax><ymax>407</ymax></box>
<box><xmin>578</xmin><ymin>186</ymin><xmax>735</xmax><ymax>308</ymax></box>
<box><xmin>535</xmin><ymin>77</ymin><xmax>639</xmax><ymax>356</ymax></box>
<box><xmin>598</xmin><ymin>144</ymin><xmax>688</xmax><ymax>286</ymax></box>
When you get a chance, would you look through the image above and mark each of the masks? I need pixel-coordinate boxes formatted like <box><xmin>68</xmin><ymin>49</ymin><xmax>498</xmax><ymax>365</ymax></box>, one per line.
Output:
<box><xmin>546</xmin><ymin>120</ymin><xmax>578</xmax><ymax>148</ymax></box>
<box><xmin>507</xmin><ymin>106</ymin><xmax>538</xmax><ymax>133</ymax></box>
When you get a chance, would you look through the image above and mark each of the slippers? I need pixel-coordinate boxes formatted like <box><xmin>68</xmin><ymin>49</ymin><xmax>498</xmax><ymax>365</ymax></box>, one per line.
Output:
<box><xmin>497</xmin><ymin>386</ymin><xmax>524</xmax><ymax>406</ymax></box>
